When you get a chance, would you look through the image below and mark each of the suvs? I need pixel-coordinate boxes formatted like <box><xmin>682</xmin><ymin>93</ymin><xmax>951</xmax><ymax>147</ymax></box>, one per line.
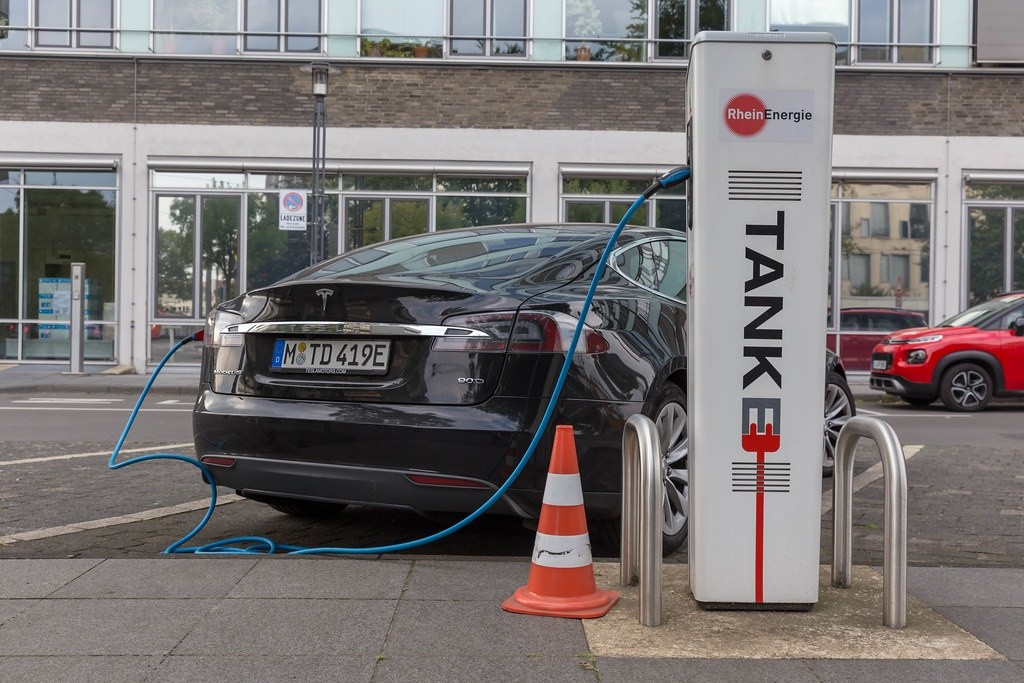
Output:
<box><xmin>869</xmin><ymin>290</ymin><xmax>1024</xmax><ymax>413</ymax></box>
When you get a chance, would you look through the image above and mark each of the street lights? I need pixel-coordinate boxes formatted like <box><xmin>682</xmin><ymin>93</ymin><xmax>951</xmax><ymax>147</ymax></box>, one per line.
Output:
<box><xmin>310</xmin><ymin>63</ymin><xmax>329</xmax><ymax>268</ymax></box>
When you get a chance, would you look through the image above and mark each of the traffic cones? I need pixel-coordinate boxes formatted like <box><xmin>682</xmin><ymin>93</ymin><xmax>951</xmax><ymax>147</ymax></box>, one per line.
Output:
<box><xmin>503</xmin><ymin>424</ymin><xmax>619</xmax><ymax>618</ymax></box>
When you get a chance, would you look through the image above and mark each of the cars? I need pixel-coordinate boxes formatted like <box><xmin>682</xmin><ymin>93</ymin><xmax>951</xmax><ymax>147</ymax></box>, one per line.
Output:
<box><xmin>827</xmin><ymin>308</ymin><xmax>930</xmax><ymax>372</ymax></box>
<box><xmin>193</xmin><ymin>222</ymin><xmax>861</xmax><ymax>556</ymax></box>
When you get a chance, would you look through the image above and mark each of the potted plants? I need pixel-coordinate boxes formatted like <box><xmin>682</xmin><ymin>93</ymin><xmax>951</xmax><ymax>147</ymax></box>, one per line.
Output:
<box><xmin>408</xmin><ymin>40</ymin><xmax>432</xmax><ymax>58</ymax></box>
<box><xmin>567</xmin><ymin>0</ymin><xmax>602</xmax><ymax>62</ymax></box>
<box><xmin>362</xmin><ymin>37</ymin><xmax>390</xmax><ymax>57</ymax></box>
